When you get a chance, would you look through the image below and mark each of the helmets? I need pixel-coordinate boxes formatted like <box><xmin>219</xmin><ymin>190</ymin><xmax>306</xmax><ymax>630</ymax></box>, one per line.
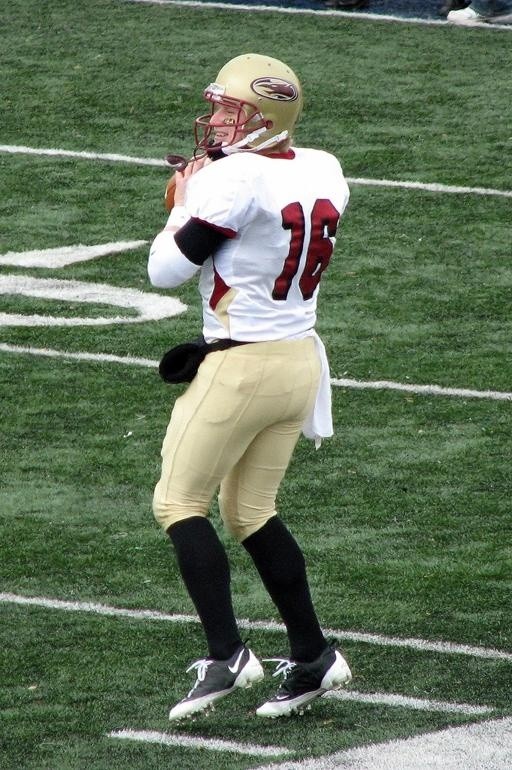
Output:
<box><xmin>195</xmin><ymin>53</ymin><xmax>303</xmax><ymax>150</ymax></box>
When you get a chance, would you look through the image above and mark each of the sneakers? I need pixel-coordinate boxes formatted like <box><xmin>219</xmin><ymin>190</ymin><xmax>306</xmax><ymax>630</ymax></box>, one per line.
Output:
<box><xmin>448</xmin><ymin>7</ymin><xmax>482</xmax><ymax>23</ymax></box>
<box><xmin>256</xmin><ymin>638</ymin><xmax>352</xmax><ymax>718</ymax></box>
<box><xmin>169</xmin><ymin>638</ymin><xmax>264</xmax><ymax>721</ymax></box>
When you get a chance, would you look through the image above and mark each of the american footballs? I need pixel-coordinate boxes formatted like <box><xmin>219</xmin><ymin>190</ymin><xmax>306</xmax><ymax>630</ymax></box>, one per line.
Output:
<box><xmin>164</xmin><ymin>168</ymin><xmax>185</xmax><ymax>211</ymax></box>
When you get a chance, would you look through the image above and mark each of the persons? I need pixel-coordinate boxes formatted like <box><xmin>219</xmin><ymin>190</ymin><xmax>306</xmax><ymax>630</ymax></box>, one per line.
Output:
<box><xmin>147</xmin><ymin>51</ymin><xmax>353</xmax><ymax>725</ymax></box>
<box><xmin>446</xmin><ymin>0</ymin><xmax>512</xmax><ymax>24</ymax></box>
